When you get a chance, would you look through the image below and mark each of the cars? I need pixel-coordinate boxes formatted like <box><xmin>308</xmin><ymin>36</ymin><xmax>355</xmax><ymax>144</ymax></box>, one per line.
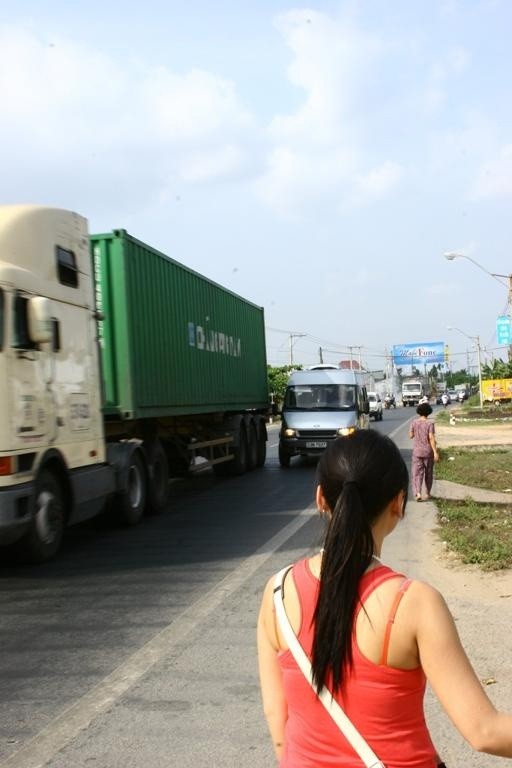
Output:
<box><xmin>436</xmin><ymin>382</ymin><xmax>478</xmax><ymax>405</ymax></box>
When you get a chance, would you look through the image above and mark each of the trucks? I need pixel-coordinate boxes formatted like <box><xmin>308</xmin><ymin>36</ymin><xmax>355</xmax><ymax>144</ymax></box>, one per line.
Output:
<box><xmin>0</xmin><ymin>200</ymin><xmax>271</xmax><ymax>569</ymax></box>
<box><xmin>478</xmin><ymin>378</ymin><xmax>512</xmax><ymax>405</ymax></box>
<box><xmin>402</xmin><ymin>381</ymin><xmax>425</xmax><ymax>406</ymax></box>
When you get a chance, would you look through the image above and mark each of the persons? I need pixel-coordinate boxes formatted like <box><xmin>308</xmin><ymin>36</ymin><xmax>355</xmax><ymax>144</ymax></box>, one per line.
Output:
<box><xmin>256</xmin><ymin>429</ymin><xmax>511</xmax><ymax>768</ymax></box>
<box><xmin>407</xmin><ymin>403</ymin><xmax>440</xmax><ymax>502</ymax></box>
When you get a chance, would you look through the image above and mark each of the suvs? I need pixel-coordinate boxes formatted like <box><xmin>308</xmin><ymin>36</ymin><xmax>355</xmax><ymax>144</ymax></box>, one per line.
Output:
<box><xmin>367</xmin><ymin>391</ymin><xmax>383</xmax><ymax>419</ymax></box>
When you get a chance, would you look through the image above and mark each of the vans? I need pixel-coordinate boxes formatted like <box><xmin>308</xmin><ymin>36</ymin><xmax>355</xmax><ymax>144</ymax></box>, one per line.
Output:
<box><xmin>279</xmin><ymin>362</ymin><xmax>372</xmax><ymax>465</ymax></box>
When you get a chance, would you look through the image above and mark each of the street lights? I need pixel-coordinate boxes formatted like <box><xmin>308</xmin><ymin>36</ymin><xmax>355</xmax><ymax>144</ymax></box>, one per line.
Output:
<box><xmin>442</xmin><ymin>250</ymin><xmax>512</xmax><ymax>315</ymax></box>
<box><xmin>449</xmin><ymin>326</ymin><xmax>484</xmax><ymax>409</ymax></box>
